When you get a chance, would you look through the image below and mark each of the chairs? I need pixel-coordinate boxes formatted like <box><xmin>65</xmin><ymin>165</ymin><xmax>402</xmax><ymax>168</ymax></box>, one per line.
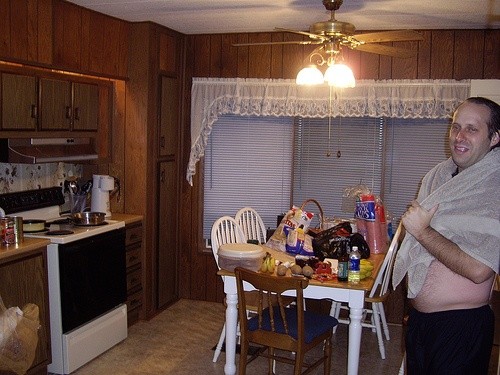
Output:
<box><xmin>211</xmin><ymin>208</ymin><xmax>402</xmax><ymax>375</ymax></box>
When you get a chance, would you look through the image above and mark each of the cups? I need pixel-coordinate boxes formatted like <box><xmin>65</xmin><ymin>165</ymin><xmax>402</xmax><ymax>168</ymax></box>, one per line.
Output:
<box><xmin>356</xmin><ymin>194</ymin><xmax>388</xmax><ymax>254</ymax></box>
<box><xmin>68</xmin><ymin>193</ymin><xmax>87</xmax><ymax>214</ymax></box>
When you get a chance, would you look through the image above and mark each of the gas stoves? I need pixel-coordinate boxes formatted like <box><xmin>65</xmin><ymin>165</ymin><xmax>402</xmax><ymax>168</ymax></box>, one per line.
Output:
<box><xmin>0</xmin><ymin>186</ymin><xmax>126</xmax><ymax>244</ymax></box>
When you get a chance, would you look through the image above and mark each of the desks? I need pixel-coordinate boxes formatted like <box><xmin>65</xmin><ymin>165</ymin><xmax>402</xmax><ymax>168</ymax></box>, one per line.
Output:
<box><xmin>217</xmin><ymin>244</ymin><xmax>389</xmax><ymax>375</ymax></box>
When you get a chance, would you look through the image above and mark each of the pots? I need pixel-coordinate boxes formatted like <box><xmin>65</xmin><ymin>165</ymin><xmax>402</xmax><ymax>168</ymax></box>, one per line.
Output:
<box><xmin>67</xmin><ymin>212</ymin><xmax>106</xmax><ymax>225</ymax></box>
<box><xmin>23</xmin><ymin>219</ymin><xmax>45</xmax><ymax>231</ymax></box>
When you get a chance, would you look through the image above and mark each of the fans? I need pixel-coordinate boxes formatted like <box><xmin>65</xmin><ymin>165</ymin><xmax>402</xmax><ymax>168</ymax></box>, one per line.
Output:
<box><xmin>233</xmin><ymin>0</ymin><xmax>425</xmax><ymax>60</ymax></box>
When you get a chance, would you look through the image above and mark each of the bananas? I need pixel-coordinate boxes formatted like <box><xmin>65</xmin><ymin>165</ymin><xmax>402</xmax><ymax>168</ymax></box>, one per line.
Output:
<box><xmin>261</xmin><ymin>252</ymin><xmax>275</xmax><ymax>273</ymax></box>
<box><xmin>360</xmin><ymin>259</ymin><xmax>374</xmax><ymax>279</ymax></box>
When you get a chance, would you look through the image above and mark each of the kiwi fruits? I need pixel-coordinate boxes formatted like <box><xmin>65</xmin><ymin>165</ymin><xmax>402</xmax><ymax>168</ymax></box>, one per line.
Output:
<box><xmin>303</xmin><ymin>266</ymin><xmax>313</xmax><ymax>276</ymax></box>
<box><xmin>291</xmin><ymin>265</ymin><xmax>302</xmax><ymax>274</ymax></box>
<box><xmin>277</xmin><ymin>265</ymin><xmax>286</xmax><ymax>275</ymax></box>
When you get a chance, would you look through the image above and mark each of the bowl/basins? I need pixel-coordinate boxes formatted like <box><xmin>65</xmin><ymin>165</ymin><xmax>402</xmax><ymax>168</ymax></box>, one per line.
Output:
<box><xmin>216</xmin><ymin>243</ymin><xmax>266</xmax><ymax>273</ymax></box>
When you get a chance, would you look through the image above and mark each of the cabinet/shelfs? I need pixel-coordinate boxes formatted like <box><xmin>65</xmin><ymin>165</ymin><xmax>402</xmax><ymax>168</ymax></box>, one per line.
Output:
<box><xmin>126</xmin><ymin>223</ymin><xmax>142</xmax><ymax>325</ymax></box>
<box><xmin>38</xmin><ymin>78</ymin><xmax>99</xmax><ymax>131</ymax></box>
<box><xmin>0</xmin><ymin>246</ymin><xmax>52</xmax><ymax>375</ymax></box>
<box><xmin>158</xmin><ymin>160</ymin><xmax>175</xmax><ymax>307</ymax></box>
<box><xmin>1</xmin><ymin>74</ymin><xmax>37</xmax><ymax>130</ymax></box>
<box><xmin>162</xmin><ymin>77</ymin><xmax>177</xmax><ymax>156</ymax></box>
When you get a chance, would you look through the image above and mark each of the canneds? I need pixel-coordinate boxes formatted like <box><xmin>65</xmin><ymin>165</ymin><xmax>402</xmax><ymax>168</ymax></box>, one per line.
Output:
<box><xmin>0</xmin><ymin>207</ymin><xmax>24</xmax><ymax>247</ymax></box>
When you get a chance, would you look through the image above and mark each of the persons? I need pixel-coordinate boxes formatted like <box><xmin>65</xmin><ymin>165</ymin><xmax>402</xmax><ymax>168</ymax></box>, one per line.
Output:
<box><xmin>391</xmin><ymin>97</ymin><xmax>500</xmax><ymax>375</ymax></box>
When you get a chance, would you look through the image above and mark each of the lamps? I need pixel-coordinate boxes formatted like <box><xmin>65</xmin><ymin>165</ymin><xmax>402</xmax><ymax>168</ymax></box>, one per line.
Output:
<box><xmin>296</xmin><ymin>43</ymin><xmax>356</xmax><ymax>158</ymax></box>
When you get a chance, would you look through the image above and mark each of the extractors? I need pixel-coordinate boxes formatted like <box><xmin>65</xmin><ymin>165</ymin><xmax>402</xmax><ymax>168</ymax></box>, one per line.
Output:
<box><xmin>0</xmin><ymin>137</ymin><xmax>99</xmax><ymax>164</ymax></box>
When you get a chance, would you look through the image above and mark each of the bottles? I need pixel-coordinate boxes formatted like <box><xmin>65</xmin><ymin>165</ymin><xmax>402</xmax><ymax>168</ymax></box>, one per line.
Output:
<box><xmin>386</xmin><ymin>211</ymin><xmax>392</xmax><ymax>242</ymax></box>
<box><xmin>337</xmin><ymin>244</ymin><xmax>349</xmax><ymax>281</ymax></box>
<box><xmin>347</xmin><ymin>246</ymin><xmax>361</xmax><ymax>285</ymax></box>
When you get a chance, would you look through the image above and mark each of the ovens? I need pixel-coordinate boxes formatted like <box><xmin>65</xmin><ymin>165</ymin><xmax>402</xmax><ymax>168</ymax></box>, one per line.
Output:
<box><xmin>47</xmin><ymin>226</ymin><xmax>127</xmax><ymax>375</ymax></box>
